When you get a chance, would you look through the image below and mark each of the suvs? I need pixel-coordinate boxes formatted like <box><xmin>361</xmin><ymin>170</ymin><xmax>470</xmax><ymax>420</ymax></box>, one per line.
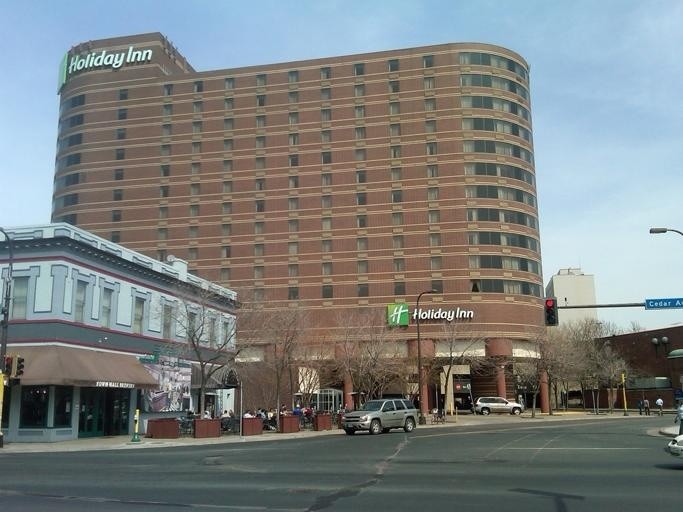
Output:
<box><xmin>473</xmin><ymin>396</ymin><xmax>524</xmax><ymax>415</ymax></box>
<box><xmin>342</xmin><ymin>398</ymin><xmax>418</xmax><ymax>435</ymax></box>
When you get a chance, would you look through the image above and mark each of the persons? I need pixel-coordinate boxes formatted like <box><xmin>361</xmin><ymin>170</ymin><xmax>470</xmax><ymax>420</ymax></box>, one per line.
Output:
<box><xmin>672</xmin><ymin>400</ymin><xmax>682</xmax><ymax>435</ymax></box>
<box><xmin>178</xmin><ymin>403</ymin><xmax>314</xmax><ymax>435</ymax></box>
<box><xmin>635</xmin><ymin>398</ymin><xmax>644</xmax><ymax>416</ymax></box>
<box><xmin>654</xmin><ymin>397</ymin><xmax>664</xmax><ymax>417</ymax></box>
<box><xmin>642</xmin><ymin>397</ymin><xmax>650</xmax><ymax>416</ymax></box>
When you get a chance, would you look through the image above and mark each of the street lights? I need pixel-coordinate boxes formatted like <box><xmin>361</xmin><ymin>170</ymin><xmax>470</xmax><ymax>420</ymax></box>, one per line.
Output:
<box><xmin>653</xmin><ymin>335</ymin><xmax>674</xmax><ymax>388</ymax></box>
<box><xmin>416</xmin><ymin>290</ymin><xmax>439</xmax><ymax>415</ymax></box>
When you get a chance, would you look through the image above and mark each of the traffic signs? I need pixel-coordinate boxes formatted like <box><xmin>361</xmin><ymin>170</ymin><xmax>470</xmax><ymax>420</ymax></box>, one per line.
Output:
<box><xmin>645</xmin><ymin>298</ymin><xmax>683</xmax><ymax>310</ymax></box>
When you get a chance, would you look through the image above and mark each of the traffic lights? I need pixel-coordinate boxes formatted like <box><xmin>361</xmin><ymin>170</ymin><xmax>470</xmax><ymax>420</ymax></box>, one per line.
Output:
<box><xmin>16</xmin><ymin>356</ymin><xmax>24</xmax><ymax>376</ymax></box>
<box><xmin>3</xmin><ymin>355</ymin><xmax>13</xmax><ymax>375</ymax></box>
<box><xmin>543</xmin><ymin>298</ymin><xmax>558</xmax><ymax>327</ymax></box>
